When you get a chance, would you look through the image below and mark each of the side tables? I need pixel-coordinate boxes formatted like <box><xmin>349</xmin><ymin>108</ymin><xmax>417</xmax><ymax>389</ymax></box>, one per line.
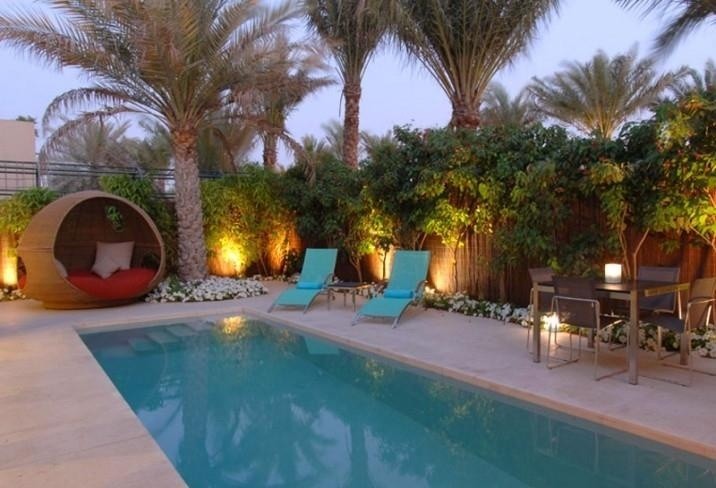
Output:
<box><xmin>326</xmin><ymin>282</ymin><xmax>373</xmax><ymax>313</ymax></box>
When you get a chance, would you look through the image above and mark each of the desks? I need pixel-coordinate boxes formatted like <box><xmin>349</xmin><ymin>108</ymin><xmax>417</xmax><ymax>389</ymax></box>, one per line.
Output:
<box><xmin>533</xmin><ymin>281</ymin><xmax>690</xmax><ymax>385</ymax></box>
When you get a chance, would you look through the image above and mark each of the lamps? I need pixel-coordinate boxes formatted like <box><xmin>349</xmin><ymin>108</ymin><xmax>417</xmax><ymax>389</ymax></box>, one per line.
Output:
<box><xmin>604</xmin><ymin>263</ymin><xmax>622</xmax><ymax>283</ymax></box>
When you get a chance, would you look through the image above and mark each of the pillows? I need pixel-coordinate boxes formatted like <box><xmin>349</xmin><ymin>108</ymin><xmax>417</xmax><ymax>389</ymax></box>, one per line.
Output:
<box><xmin>96</xmin><ymin>240</ymin><xmax>135</xmax><ymax>271</ymax></box>
<box><xmin>54</xmin><ymin>258</ymin><xmax>67</xmax><ymax>278</ymax></box>
<box><xmin>91</xmin><ymin>257</ymin><xmax>120</xmax><ymax>280</ymax></box>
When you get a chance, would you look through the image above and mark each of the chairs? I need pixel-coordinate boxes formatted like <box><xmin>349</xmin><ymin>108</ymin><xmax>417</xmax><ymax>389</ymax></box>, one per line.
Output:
<box><xmin>350</xmin><ymin>251</ymin><xmax>431</xmax><ymax>329</ymax></box>
<box><xmin>528</xmin><ymin>266</ymin><xmax>600</xmax><ymax>363</ymax></box>
<box><xmin>636</xmin><ymin>276</ymin><xmax>716</xmax><ymax>388</ymax></box>
<box><xmin>546</xmin><ymin>274</ymin><xmax>629</xmax><ymax>381</ymax></box>
<box><xmin>609</xmin><ymin>267</ymin><xmax>680</xmax><ymax>360</ymax></box>
<box><xmin>266</xmin><ymin>248</ymin><xmax>338</xmax><ymax>314</ymax></box>
